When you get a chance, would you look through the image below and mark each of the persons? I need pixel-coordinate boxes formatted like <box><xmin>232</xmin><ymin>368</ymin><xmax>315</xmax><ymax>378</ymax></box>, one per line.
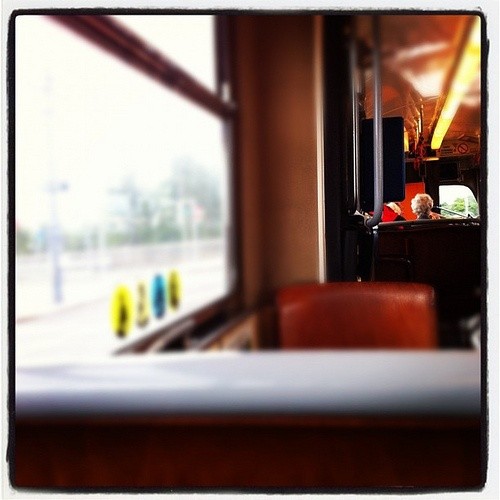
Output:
<box><xmin>410</xmin><ymin>194</ymin><xmax>434</xmax><ymax>219</ymax></box>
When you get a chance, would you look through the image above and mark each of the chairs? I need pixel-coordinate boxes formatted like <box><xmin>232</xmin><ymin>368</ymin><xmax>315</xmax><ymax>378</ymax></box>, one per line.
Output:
<box><xmin>275</xmin><ymin>280</ymin><xmax>439</xmax><ymax>354</ymax></box>
